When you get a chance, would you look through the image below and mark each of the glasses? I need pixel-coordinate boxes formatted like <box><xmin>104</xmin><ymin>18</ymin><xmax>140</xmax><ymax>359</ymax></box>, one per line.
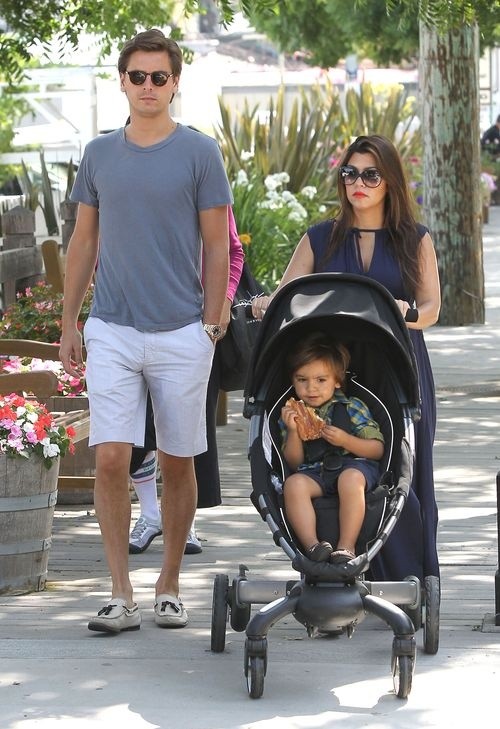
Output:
<box><xmin>338</xmin><ymin>164</ymin><xmax>383</xmax><ymax>189</ymax></box>
<box><xmin>124</xmin><ymin>67</ymin><xmax>173</xmax><ymax>87</ymax></box>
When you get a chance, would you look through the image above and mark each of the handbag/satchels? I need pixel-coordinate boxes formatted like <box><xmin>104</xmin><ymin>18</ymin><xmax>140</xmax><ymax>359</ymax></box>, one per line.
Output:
<box><xmin>211</xmin><ymin>260</ymin><xmax>271</xmax><ymax>392</ymax></box>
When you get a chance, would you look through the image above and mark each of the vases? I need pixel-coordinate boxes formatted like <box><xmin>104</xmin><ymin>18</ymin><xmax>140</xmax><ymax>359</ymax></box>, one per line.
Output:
<box><xmin>0</xmin><ymin>446</ymin><xmax>63</xmax><ymax>599</ymax></box>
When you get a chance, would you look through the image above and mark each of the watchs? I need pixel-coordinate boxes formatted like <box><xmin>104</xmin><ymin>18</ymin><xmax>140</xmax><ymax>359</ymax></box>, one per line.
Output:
<box><xmin>203</xmin><ymin>324</ymin><xmax>221</xmax><ymax>339</ymax></box>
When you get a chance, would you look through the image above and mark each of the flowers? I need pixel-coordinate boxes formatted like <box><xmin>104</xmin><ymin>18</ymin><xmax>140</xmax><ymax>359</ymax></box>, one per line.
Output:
<box><xmin>0</xmin><ymin>280</ymin><xmax>90</xmax><ymax>468</ymax></box>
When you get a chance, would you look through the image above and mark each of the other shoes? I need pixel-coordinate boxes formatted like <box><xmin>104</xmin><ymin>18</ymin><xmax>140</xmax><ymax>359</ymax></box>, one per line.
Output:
<box><xmin>306</xmin><ymin>540</ymin><xmax>333</xmax><ymax>562</ymax></box>
<box><xmin>331</xmin><ymin>548</ymin><xmax>356</xmax><ymax>564</ymax></box>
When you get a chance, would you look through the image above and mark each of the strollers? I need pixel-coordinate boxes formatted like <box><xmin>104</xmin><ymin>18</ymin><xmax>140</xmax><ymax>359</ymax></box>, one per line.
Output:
<box><xmin>210</xmin><ymin>271</ymin><xmax>440</xmax><ymax>704</ymax></box>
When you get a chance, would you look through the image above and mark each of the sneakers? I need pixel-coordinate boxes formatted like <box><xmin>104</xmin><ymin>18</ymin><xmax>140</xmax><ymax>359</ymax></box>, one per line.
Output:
<box><xmin>88</xmin><ymin>602</ymin><xmax>142</xmax><ymax>632</ymax></box>
<box><xmin>129</xmin><ymin>510</ymin><xmax>163</xmax><ymax>554</ymax></box>
<box><xmin>184</xmin><ymin>520</ymin><xmax>203</xmax><ymax>553</ymax></box>
<box><xmin>154</xmin><ymin>594</ymin><xmax>188</xmax><ymax>627</ymax></box>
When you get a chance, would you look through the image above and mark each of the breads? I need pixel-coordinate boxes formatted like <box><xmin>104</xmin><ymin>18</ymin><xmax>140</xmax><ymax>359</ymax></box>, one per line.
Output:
<box><xmin>285</xmin><ymin>397</ymin><xmax>326</xmax><ymax>441</ymax></box>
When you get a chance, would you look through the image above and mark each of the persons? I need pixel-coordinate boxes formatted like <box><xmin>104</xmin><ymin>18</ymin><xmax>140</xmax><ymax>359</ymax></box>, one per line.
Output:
<box><xmin>57</xmin><ymin>31</ymin><xmax>244</xmax><ymax>632</ymax></box>
<box><xmin>276</xmin><ymin>332</ymin><xmax>386</xmax><ymax>564</ymax></box>
<box><xmin>251</xmin><ymin>134</ymin><xmax>442</xmax><ymax>576</ymax></box>
